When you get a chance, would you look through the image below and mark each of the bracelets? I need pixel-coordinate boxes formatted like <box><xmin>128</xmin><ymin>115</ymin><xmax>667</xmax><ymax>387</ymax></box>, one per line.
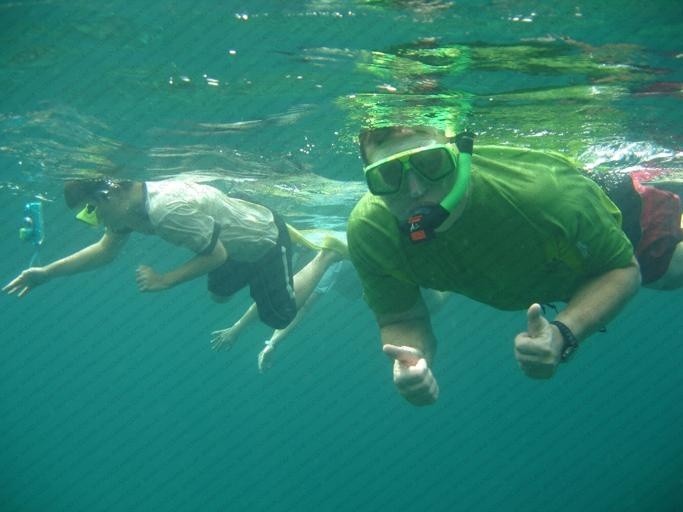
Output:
<box><xmin>265</xmin><ymin>338</ymin><xmax>276</xmax><ymax>350</ymax></box>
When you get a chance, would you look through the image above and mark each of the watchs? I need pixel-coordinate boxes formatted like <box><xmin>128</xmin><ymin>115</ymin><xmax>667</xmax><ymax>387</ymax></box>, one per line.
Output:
<box><xmin>553</xmin><ymin>318</ymin><xmax>578</xmax><ymax>366</ymax></box>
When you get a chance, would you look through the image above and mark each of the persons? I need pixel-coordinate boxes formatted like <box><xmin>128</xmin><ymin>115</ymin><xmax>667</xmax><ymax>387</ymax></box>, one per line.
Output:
<box><xmin>343</xmin><ymin>123</ymin><xmax>683</xmax><ymax>407</ymax></box>
<box><xmin>0</xmin><ymin>174</ymin><xmax>351</xmax><ymax>328</ymax></box>
<box><xmin>206</xmin><ymin>247</ymin><xmax>448</xmax><ymax>371</ymax></box>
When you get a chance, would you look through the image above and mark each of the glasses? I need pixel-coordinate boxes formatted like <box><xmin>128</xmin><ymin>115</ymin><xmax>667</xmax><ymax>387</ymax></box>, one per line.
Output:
<box><xmin>76</xmin><ymin>200</ymin><xmax>106</xmax><ymax>230</ymax></box>
<box><xmin>363</xmin><ymin>143</ymin><xmax>461</xmax><ymax>196</ymax></box>
